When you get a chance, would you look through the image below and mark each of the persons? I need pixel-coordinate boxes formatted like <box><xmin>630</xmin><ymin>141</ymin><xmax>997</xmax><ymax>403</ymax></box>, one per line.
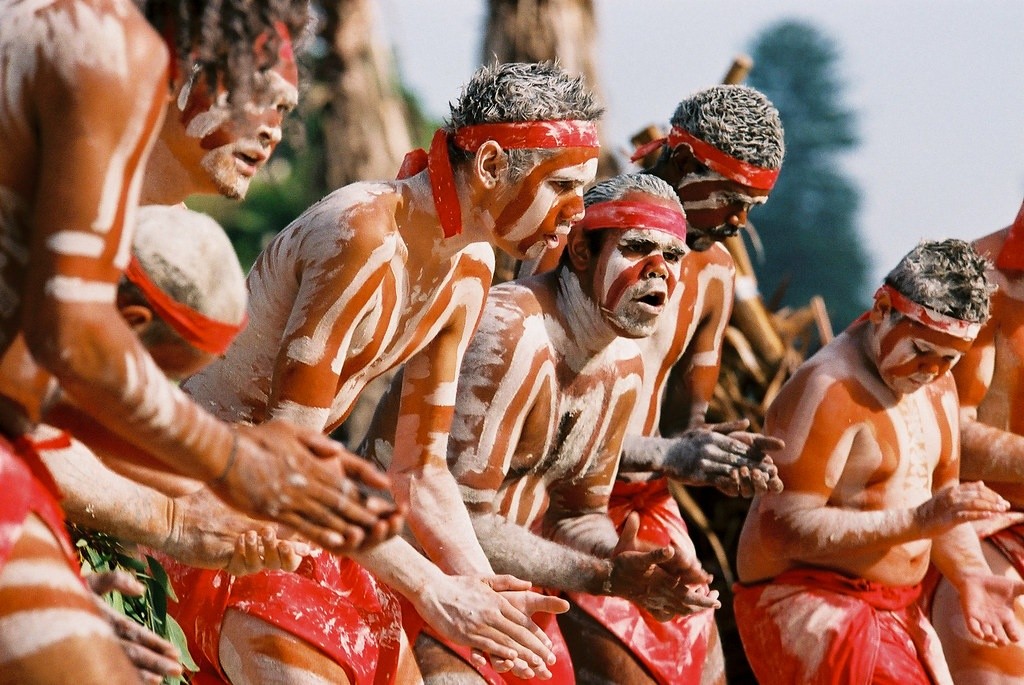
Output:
<box><xmin>0</xmin><ymin>0</ymin><xmax>1024</xmax><ymax>685</ymax></box>
